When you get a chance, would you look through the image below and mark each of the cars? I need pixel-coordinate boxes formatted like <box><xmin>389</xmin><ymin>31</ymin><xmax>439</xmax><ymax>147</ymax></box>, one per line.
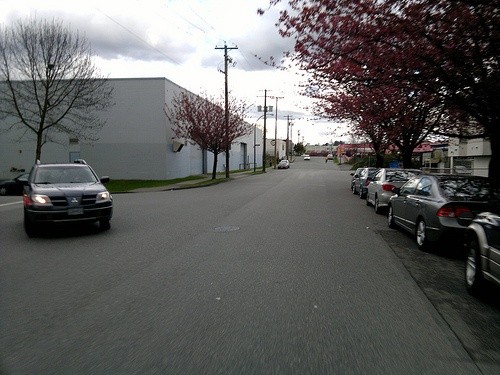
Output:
<box><xmin>277</xmin><ymin>159</ymin><xmax>290</xmax><ymax>169</ymax></box>
<box><xmin>462</xmin><ymin>206</ymin><xmax>500</xmax><ymax>298</ymax></box>
<box><xmin>303</xmin><ymin>154</ymin><xmax>311</xmax><ymax>160</ymax></box>
<box><xmin>366</xmin><ymin>168</ymin><xmax>424</xmax><ymax>215</ymax></box>
<box><xmin>350</xmin><ymin>167</ymin><xmax>379</xmax><ymax>199</ymax></box>
<box><xmin>0</xmin><ymin>172</ymin><xmax>29</xmax><ymax>196</ymax></box>
<box><xmin>387</xmin><ymin>172</ymin><xmax>500</xmax><ymax>254</ymax></box>
<box><xmin>326</xmin><ymin>154</ymin><xmax>333</xmax><ymax>160</ymax></box>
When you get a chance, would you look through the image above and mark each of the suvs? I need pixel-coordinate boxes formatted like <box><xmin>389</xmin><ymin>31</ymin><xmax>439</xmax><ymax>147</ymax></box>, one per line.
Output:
<box><xmin>16</xmin><ymin>157</ymin><xmax>114</xmax><ymax>238</ymax></box>
